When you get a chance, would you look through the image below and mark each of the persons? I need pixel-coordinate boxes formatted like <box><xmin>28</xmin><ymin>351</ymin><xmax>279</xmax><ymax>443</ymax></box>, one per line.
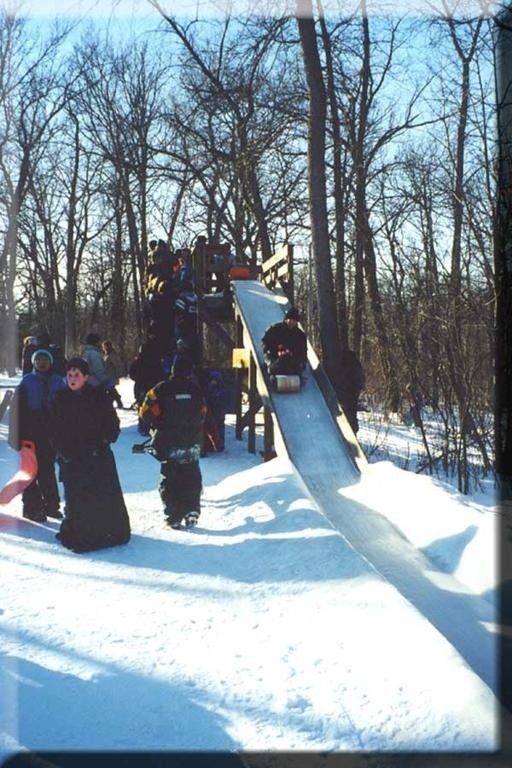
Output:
<box><xmin>143</xmin><ymin>236</ymin><xmax>252</xmax><ymax>338</ymax></box>
<box><xmin>261</xmin><ymin>308</ymin><xmax>307</xmax><ymax>383</ymax></box>
<box><xmin>10</xmin><ymin>325</ymin><xmax>225</xmax><ymax>552</ymax></box>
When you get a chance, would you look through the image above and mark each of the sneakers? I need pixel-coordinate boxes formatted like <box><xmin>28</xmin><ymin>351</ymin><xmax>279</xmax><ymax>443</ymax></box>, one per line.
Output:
<box><xmin>47</xmin><ymin>509</ymin><xmax>63</xmax><ymax>518</ymax></box>
<box><xmin>185</xmin><ymin>510</ymin><xmax>198</xmax><ymax>527</ymax></box>
<box><xmin>171</xmin><ymin>522</ymin><xmax>181</xmax><ymax>529</ymax></box>
<box><xmin>23</xmin><ymin>510</ymin><xmax>46</xmax><ymax>522</ymax></box>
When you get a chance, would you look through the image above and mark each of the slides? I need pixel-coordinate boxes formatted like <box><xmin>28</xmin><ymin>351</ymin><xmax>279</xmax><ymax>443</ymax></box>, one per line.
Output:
<box><xmin>229</xmin><ymin>276</ymin><xmax>498</xmax><ymax>692</ymax></box>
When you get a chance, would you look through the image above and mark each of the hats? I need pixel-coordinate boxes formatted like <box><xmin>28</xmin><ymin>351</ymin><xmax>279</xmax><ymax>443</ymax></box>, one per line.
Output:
<box><xmin>285</xmin><ymin>309</ymin><xmax>301</xmax><ymax>322</ymax></box>
<box><xmin>172</xmin><ymin>355</ymin><xmax>192</xmax><ymax>377</ymax></box>
<box><xmin>30</xmin><ymin>349</ymin><xmax>53</xmax><ymax>368</ymax></box>
<box><xmin>66</xmin><ymin>357</ymin><xmax>89</xmax><ymax>373</ymax></box>
